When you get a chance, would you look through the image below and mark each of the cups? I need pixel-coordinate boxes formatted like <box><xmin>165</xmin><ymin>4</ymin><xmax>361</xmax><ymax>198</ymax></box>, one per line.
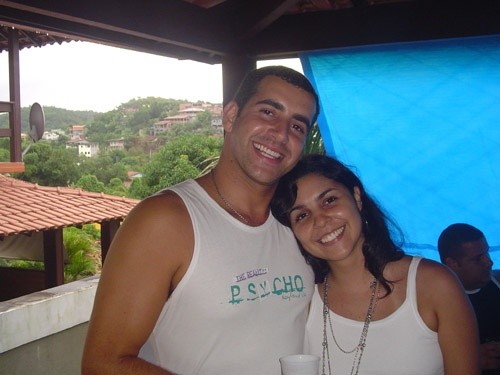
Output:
<box><xmin>279</xmin><ymin>354</ymin><xmax>320</xmax><ymax>375</ymax></box>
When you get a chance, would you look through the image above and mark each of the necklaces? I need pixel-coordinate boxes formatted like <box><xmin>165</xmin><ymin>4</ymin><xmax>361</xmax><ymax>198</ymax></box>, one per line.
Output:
<box><xmin>211</xmin><ymin>168</ymin><xmax>258</xmax><ymax>226</ymax></box>
<box><xmin>320</xmin><ymin>262</ymin><xmax>387</xmax><ymax>374</ymax></box>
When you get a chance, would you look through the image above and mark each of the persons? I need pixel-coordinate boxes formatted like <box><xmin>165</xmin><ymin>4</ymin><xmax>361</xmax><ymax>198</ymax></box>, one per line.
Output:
<box><xmin>437</xmin><ymin>222</ymin><xmax>500</xmax><ymax>375</ymax></box>
<box><xmin>82</xmin><ymin>66</ymin><xmax>320</xmax><ymax>375</ymax></box>
<box><xmin>272</xmin><ymin>154</ymin><xmax>481</xmax><ymax>375</ymax></box>
